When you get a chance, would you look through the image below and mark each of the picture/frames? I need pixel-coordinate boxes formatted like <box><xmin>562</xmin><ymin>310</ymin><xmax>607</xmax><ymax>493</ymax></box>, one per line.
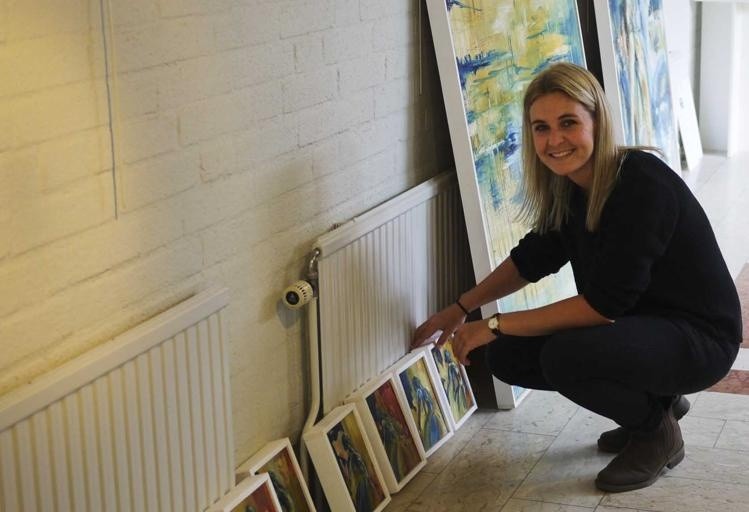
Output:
<box><xmin>411</xmin><ymin>329</ymin><xmax>478</xmax><ymax>431</ymax></box>
<box><xmin>235</xmin><ymin>437</ymin><xmax>317</xmax><ymax>512</ymax></box>
<box><xmin>343</xmin><ymin>371</ymin><xmax>427</xmax><ymax>494</ymax></box>
<box><xmin>593</xmin><ymin>0</ymin><xmax>682</xmax><ymax>182</ymax></box>
<box><xmin>388</xmin><ymin>350</ymin><xmax>455</xmax><ymax>458</ymax></box>
<box><xmin>204</xmin><ymin>472</ymin><xmax>283</xmax><ymax>512</ymax></box>
<box><xmin>301</xmin><ymin>402</ymin><xmax>391</xmax><ymax>512</ymax></box>
<box><xmin>423</xmin><ymin>0</ymin><xmax>589</xmax><ymax>409</ymax></box>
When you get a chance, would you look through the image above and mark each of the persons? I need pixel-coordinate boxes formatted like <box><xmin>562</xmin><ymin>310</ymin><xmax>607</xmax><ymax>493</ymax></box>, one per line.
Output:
<box><xmin>409</xmin><ymin>63</ymin><xmax>742</xmax><ymax>495</ymax></box>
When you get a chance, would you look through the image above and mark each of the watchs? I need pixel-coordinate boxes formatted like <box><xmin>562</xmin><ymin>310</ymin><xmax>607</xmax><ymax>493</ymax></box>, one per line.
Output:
<box><xmin>487</xmin><ymin>312</ymin><xmax>504</xmax><ymax>339</ymax></box>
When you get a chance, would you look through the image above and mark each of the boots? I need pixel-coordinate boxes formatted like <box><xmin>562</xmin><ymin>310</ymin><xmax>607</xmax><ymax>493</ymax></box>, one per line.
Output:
<box><xmin>596</xmin><ymin>395</ymin><xmax>690</xmax><ymax>454</ymax></box>
<box><xmin>595</xmin><ymin>411</ymin><xmax>685</xmax><ymax>493</ymax></box>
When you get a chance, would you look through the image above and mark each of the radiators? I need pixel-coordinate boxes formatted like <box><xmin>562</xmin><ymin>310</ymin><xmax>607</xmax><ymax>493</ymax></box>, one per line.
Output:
<box><xmin>281</xmin><ymin>167</ymin><xmax>477</xmax><ymax>417</ymax></box>
<box><xmin>0</xmin><ymin>285</ymin><xmax>237</xmax><ymax>512</ymax></box>
